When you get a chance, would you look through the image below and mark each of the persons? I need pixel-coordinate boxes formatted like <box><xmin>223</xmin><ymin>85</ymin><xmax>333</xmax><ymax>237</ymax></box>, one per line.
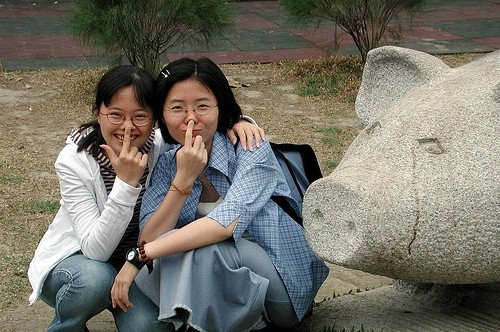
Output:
<box><xmin>111</xmin><ymin>57</ymin><xmax>331</xmax><ymax>332</ymax></box>
<box><xmin>27</xmin><ymin>66</ymin><xmax>265</xmax><ymax>332</ymax></box>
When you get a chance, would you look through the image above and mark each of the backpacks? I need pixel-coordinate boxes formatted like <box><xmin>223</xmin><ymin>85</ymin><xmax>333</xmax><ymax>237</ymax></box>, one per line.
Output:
<box><xmin>234</xmin><ymin>136</ymin><xmax>324</xmax><ymax>228</ymax></box>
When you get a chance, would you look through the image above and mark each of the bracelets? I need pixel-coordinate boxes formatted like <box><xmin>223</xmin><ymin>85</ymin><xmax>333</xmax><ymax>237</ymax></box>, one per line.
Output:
<box><xmin>138</xmin><ymin>240</ymin><xmax>152</xmax><ymax>263</ymax></box>
<box><xmin>171</xmin><ymin>182</ymin><xmax>192</xmax><ymax>195</ymax></box>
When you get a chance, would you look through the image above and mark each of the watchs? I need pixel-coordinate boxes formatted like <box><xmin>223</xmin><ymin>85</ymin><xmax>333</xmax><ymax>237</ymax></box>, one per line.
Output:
<box><xmin>125</xmin><ymin>247</ymin><xmax>144</xmax><ymax>269</ymax></box>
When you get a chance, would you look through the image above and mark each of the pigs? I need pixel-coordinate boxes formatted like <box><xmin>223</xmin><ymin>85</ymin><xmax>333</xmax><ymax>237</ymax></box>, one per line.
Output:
<box><xmin>300</xmin><ymin>43</ymin><xmax>500</xmax><ymax>287</ymax></box>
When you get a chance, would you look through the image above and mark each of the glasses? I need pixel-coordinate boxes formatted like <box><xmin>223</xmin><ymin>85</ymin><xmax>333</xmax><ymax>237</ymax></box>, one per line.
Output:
<box><xmin>98</xmin><ymin>111</ymin><xmax>150</xmax><ymax>127</ymax></box>
<box><xmin>163</xmin><ymin>102</ymin><xmax>218</xmax><ymax>116</ymax></box>
